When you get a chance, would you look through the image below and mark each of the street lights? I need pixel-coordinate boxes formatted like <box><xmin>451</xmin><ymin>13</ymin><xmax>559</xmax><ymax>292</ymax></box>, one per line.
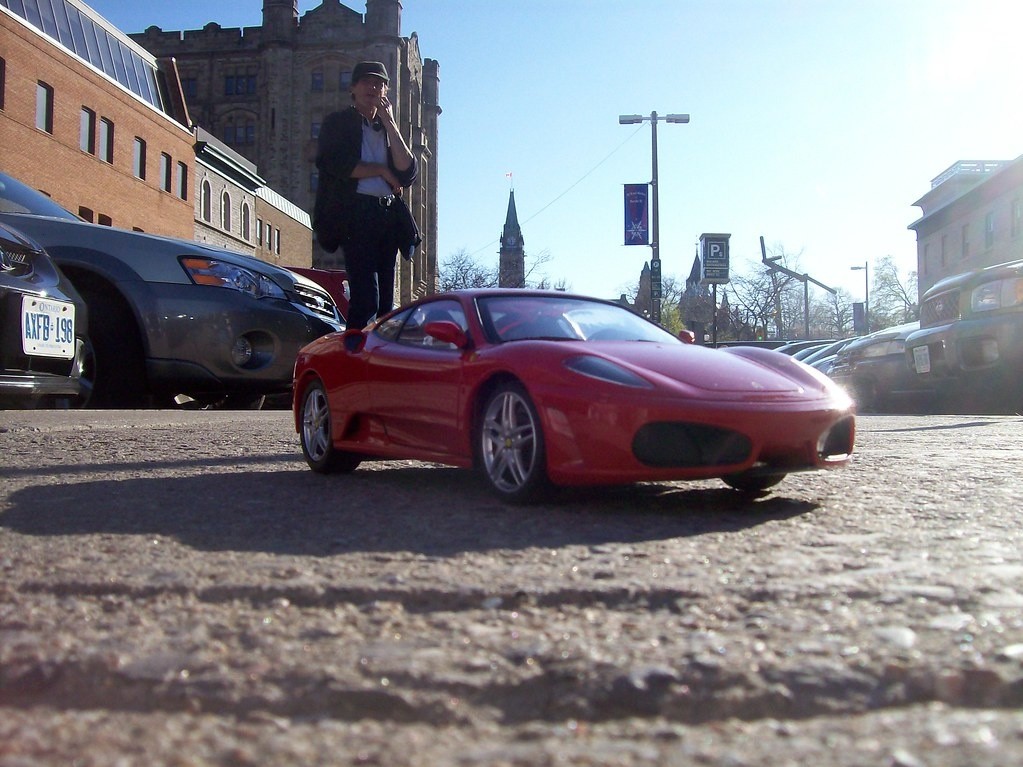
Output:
<box><xmin>850</xmin><ymin>260</ymin><xmax>869</xmax><ymax>335</ymax></box>
<box><xmin>619</xmin><ymin>112</ymin><xmax>689</xmax><ymax>325</ymax></box>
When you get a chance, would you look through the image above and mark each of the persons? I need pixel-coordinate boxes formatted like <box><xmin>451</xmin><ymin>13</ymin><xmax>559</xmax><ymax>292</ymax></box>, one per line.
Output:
<box><xmin>311</xmin><ymin>61</ymin><xmax>422</xmax><ymax>330</ymax></box>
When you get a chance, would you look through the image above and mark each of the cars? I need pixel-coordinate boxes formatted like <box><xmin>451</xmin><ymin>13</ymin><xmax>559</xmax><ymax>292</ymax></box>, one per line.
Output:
<box><xmin>0</xmin><ymin>220</ymin><xmax>96</xmax><ymax>408</ymax></box>
<box><xmin>765</xmin><ymin>257</ymin><xmax>1023</xmax><ymax>409</ymax></box>
<box><xmin>0</xmin><ymin>172</ymin><xmax>346</xmax><ymax>408</ymax></box>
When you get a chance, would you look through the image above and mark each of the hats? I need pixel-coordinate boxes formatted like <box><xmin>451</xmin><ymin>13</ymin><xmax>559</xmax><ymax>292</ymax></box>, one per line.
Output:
<box><xmin>351</xmin><ymin>62</ymin><xmax>390</xmax><ymax>87</ymax></box>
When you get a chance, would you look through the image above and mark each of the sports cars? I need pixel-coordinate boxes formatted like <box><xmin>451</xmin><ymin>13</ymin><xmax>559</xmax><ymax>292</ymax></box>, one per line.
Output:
<box><xmin>288</xmin><ymin>285</ymin><xmax>859</xmax><ymax>507</ymax></box>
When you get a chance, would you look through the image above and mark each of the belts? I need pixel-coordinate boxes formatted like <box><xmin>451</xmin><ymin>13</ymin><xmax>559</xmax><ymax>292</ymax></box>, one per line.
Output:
<box><xmin>352</xmin><ymin>193</ymin><xmax>397</xmax><ymax>208</ymax></box>
